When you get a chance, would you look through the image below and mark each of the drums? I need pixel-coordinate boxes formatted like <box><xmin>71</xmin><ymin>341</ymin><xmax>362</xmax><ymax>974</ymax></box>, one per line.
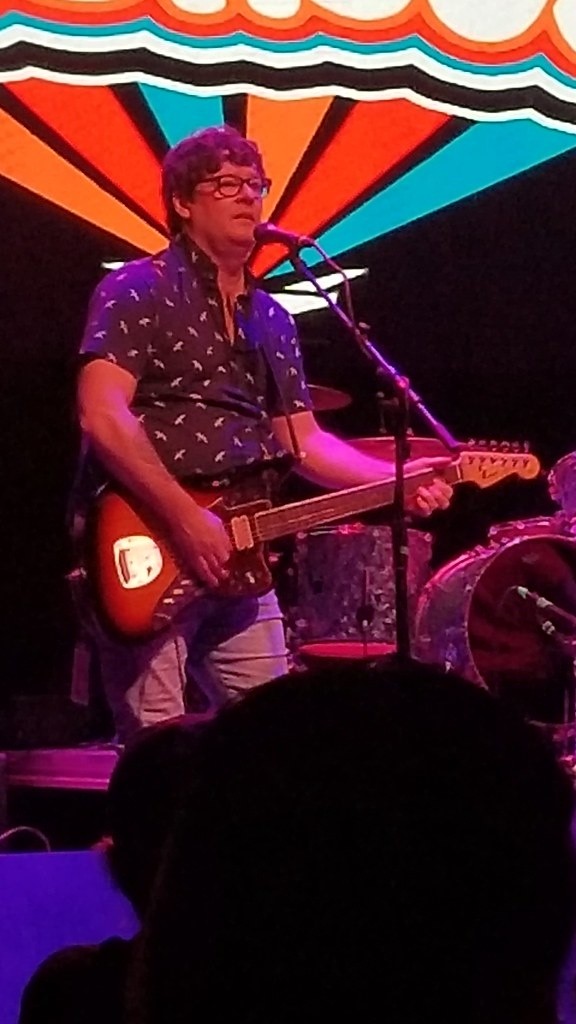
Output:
<box><xmin>270</xmin><ymin>523</ymin><xmax>435</xmax><ymax>660</ymax></box>
<box><xmin>410</xmin><ymin>532</ymin><xmax>576</xmax><ymax>742</ymax></box>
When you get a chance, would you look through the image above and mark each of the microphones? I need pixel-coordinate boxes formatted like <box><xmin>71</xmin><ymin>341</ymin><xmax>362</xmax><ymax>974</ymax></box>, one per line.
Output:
<box><xmin>253</xmin><ymin>222</ymin><xmax>315</xmax><ymax>248</ymax></box>
<box><xmin>516</xmin><ymin>586</ymin><xmax>576</xmax><ymax>631</ymax></box>
<box><xmin>536</xmin><ymin>614</ymin><xmax>576</xmax><ymax>666</ymax></box>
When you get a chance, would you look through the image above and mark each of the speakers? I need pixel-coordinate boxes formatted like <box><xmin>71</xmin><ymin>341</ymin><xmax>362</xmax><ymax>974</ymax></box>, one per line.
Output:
<box><xmin>0</xmin><ymin>849</ymin><xmax>142</xmax><ymax>1024</ymax></box>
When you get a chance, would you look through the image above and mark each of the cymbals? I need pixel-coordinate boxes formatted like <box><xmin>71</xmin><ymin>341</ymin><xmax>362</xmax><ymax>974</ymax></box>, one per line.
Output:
<box><xmin>305</xmin><ymin>383</ymin><xmax>354</xmax><ymax>413</ymax></box>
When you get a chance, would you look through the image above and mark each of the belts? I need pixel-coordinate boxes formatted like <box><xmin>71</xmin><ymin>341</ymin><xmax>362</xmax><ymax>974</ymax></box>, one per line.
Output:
<box><xmin>184</xmin><ymin>464</ymin><xmax>263</xmax><ymax>497</ymax></box>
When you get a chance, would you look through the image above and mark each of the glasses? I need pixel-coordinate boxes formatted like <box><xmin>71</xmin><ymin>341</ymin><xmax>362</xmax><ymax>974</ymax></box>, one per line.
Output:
<box><xmin>187</xmin><ymin>173</ymin><xmax>271</xmax><ymax>198</ymax></box>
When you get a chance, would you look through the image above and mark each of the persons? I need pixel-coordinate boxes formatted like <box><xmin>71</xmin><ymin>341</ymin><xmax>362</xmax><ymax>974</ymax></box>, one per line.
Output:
<box><xmin>17</xmin><ymin>659</ymin><xmax>576</xmax><ymax>1024</ymax></box>
<box><xmin>75</xmin><ymin>122</ymin><xmax>453</xmax><ymax>741</ymax></box>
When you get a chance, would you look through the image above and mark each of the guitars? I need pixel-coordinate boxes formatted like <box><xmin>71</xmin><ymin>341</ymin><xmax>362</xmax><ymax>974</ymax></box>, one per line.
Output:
<box><xmin>88</xmin><ymin>438</ymin><xmax>546</xmax><ymax>640</ymax></box>
